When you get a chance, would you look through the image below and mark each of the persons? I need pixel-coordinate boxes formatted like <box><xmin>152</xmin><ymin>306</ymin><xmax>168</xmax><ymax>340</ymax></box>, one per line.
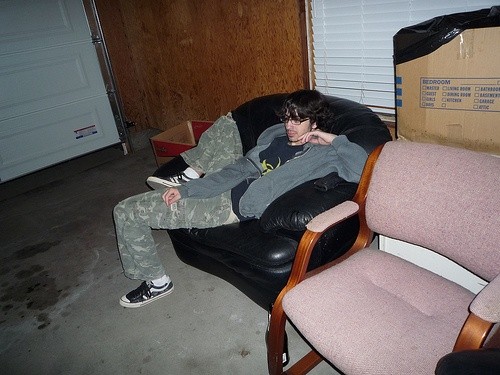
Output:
<box><xmin>113</xmin><ymin>90</ymin><xmax>368</xmax><ymax>308</ymax></box>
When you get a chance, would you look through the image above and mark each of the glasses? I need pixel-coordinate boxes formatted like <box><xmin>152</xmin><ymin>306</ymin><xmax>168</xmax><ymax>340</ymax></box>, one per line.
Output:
<box><xmin>281</xmin><ymin>114</ymin><xmax>310</xmax><ymax>125</ymax></box>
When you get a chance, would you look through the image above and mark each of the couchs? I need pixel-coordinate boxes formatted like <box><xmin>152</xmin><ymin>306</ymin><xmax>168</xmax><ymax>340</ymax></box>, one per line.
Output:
<box><xmin>150</xmin><ymin>92</ymin><xmax>392</xmax><ymax>315</ymax></box>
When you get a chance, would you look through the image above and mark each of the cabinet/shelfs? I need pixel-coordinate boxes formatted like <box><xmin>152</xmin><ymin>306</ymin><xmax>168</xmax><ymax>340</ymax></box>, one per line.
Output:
<box><xmin>0</xmin><ymin>0</ymin><xmax>134</xmax><ymax>186</ymax></box>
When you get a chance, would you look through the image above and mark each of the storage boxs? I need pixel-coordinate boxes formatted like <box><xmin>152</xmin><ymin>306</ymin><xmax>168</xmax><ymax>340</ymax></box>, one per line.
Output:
<box><xmin>151</xmin><ymin>121</ymin><xmax>215</xmax><ymax>168</ymax></box>
<box><xmin>394</xmin><ymin>26</ymin><xmax>500</xmax><ymax>158</ymax></box>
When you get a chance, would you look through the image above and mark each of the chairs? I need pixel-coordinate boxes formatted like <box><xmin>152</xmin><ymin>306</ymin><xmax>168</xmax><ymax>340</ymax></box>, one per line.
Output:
<box><xmin>267</xmin><ymin>140</ymin><xmax>500</xmax><ymax>375</ymax></box>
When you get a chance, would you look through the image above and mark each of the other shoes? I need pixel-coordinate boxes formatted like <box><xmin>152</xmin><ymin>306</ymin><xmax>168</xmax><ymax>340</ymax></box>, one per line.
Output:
<box><xmin>119</xmin><ymin>278</ymin><xmax>174</xmax><ymax>309</ymax></box>
<box><xmin>146</xmin><ymin>172</ymin><xmax>197</xmax><ymax>191</ymax></box>
